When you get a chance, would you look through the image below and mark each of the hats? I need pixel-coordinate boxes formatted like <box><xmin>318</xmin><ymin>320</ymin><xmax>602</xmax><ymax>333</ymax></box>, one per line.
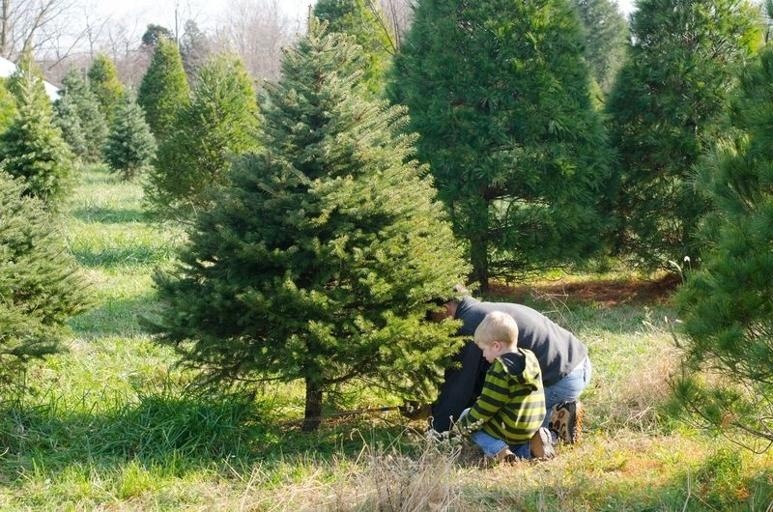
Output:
<box><xmin>424</xmin><ymin>289</ymin><xmax>464</xmax><ymax>322</ymax></box>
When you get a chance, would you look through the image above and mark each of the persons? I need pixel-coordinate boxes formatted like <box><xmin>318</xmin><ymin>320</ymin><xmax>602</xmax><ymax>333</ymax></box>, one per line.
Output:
<box><xmin>452</xmin><ymin>310</ymin><xmax>555</xmax><ymax>469</ymax></box>
<box><xmin>422</xmin><ymin>283</ymin><xmax>594</xmax><ymax>447</ymax></box>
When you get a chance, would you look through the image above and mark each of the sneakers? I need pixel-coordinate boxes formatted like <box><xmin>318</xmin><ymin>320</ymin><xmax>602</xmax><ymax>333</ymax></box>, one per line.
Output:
<box><xmin>532</xmin><ymin>428</ymin><xmax>555</xmax><ymax>458</ymax></box>
<box><xmin>550</xmin><ymin>401</ymin><xmax>582</xmax><ymax>447</ymax></box>
<box><xmin>485</xmin><ymin>448</ymin><xmax>515</xmax><ymax>468</ymax></box>
